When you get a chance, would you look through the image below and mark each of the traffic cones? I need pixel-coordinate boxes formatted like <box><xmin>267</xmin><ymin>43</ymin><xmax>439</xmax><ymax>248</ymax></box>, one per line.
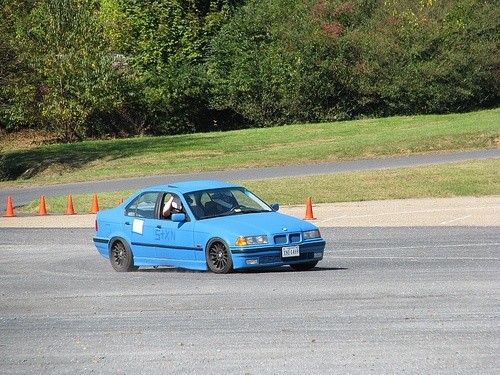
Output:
<box><xmin>2</xmin><ymin>196</ymin><xmax>17</xmax><ymax>217</ymax></box>
<box><xmin>63</xmin><ymin>195</ymin><xmax>77</xmax><ymax>215</ymax></box>
<box><xmin>302</xmin><ymin>196</ymin><xmax>317</xmax><ymax>220</ymax></box>
<box><xmin>89</xmin><ymin>194</ymin><xmax>100</xmax><ymax>214</ymax></box>
<box><xmin>35</xmin><ymin>195</ymin><xmax>49</xmax><ymax>216</ymax></box>
<box><xmin>118</xmin><ymin>198</ymin><xmax>123</xmax><ymax>205</ymax></box>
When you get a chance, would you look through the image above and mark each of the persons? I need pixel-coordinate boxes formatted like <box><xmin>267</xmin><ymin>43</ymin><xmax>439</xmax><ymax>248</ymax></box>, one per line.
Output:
<box><xmin>213</xmin><ymin>194</ymin><xmax>237</xmax><ymax>216</ymax></box>
<box><xmin>162</xmin><ymin>193</ymin><xmax>200</xmax><ymax>222</ymax></box>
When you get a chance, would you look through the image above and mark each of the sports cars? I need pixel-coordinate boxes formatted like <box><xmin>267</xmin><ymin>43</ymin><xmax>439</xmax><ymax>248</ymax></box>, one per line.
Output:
<box><xmin>93</xmin><ymin>180</ymin><xmax>326</xmax><ymax>274</ymax></box>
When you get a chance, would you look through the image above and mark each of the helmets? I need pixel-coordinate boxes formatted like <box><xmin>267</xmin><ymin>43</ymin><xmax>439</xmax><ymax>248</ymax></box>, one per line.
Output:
<box><xmin>171</xmin><ymin>194</ymin><xmax>194</xmax><ymax>210</ymax></box>
<box><xmin>212</xmin><ymin>189</ymin><xmax>233</xmax><ymax>209</ymax></box>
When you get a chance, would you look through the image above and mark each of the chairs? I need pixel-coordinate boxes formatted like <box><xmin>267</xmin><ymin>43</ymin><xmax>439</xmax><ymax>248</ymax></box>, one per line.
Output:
<box><xmin>205</xmin><ymin>201</ymin><xmax>218</xmax><ymax>216</ymax></box>
